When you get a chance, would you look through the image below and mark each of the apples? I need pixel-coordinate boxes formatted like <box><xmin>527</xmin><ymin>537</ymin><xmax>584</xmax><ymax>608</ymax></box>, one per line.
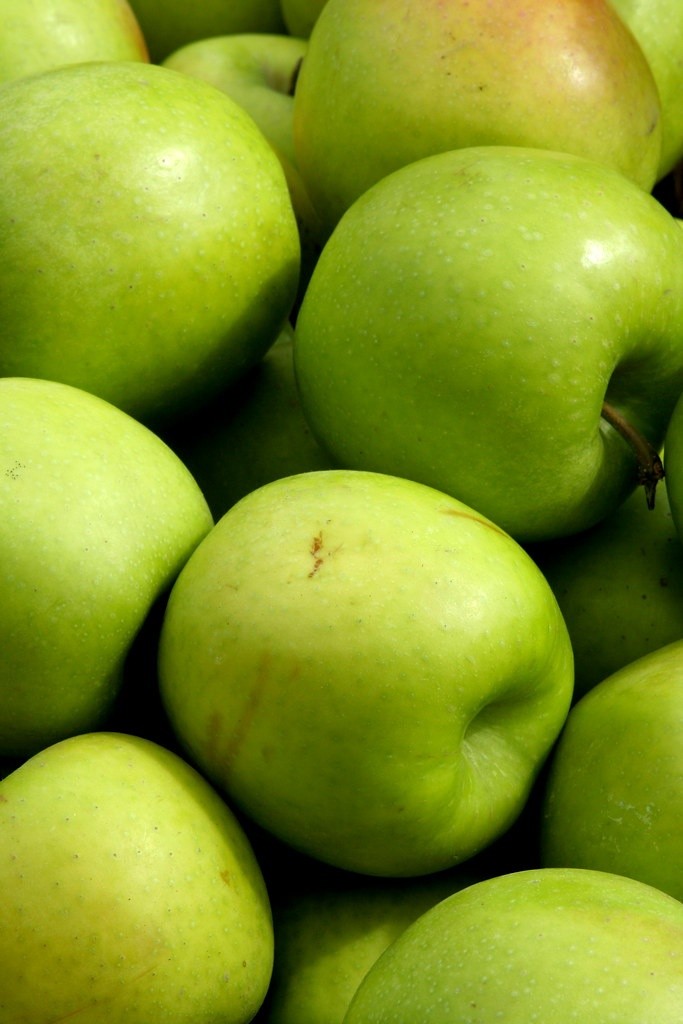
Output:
<box><xmin>0</xmin><ymin>0</ymin><xmax>683</xmax><ymax>1024</ymax></box>
